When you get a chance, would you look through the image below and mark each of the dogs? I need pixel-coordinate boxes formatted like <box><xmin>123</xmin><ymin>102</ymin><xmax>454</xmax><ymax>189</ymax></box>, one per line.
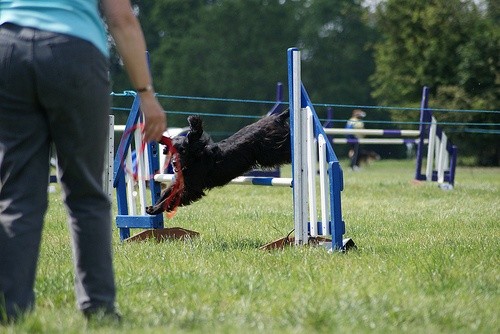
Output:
<box><xmin>146</xmin><ymin>108</ymin><xmax>291</xmax><ymax>215</ymax></box>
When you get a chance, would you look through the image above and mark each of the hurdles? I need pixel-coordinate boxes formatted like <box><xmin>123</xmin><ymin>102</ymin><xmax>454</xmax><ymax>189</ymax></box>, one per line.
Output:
<box><xmin>106</xmin><ymin>49</ymin><xmax>461</xmax><ymax>249</ymax></box>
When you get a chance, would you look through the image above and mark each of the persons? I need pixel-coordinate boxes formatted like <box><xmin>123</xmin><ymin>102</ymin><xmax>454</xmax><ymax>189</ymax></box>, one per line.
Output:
<box><xmin>0</xmin><ymin>0</ymin><xmax>167</xmax><ymax>325</ymax></box>
<box><xmin>344</xmin><ymin>110</ymin><xmax>366</xmax><ymax>171</ymax></box>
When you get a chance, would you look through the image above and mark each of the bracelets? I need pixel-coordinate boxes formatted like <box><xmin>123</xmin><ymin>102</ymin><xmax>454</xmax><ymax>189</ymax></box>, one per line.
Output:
<box><xmin>136</xmin><ymin>84</ymin><xmax>155</xmax><ymax>93</ymax></box>
